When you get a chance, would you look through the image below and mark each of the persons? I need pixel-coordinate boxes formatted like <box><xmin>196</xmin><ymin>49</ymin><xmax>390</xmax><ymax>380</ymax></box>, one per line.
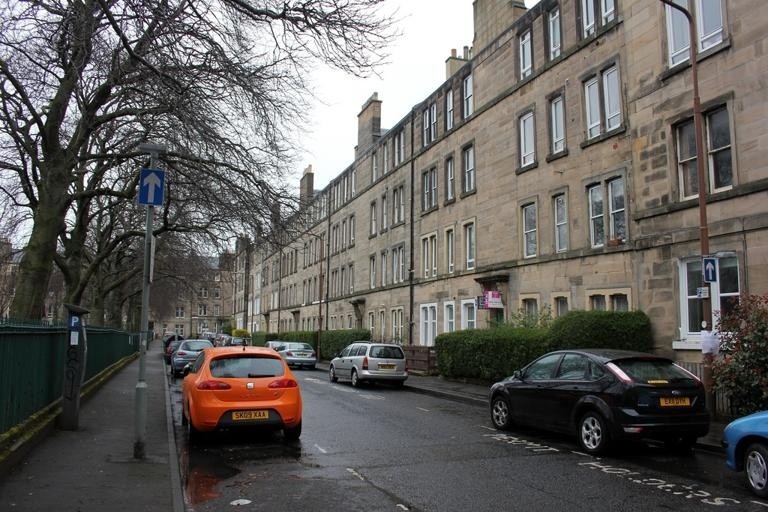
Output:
<box><xmin>242</xmin><ymin>338</ymin><xmax>248</xmax><ymax>346</ymax></box>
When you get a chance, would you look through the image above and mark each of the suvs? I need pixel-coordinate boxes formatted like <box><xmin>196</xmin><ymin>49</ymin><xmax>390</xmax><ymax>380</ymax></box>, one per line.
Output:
<box><xmin>328</xmin><ymin>340</ymin><xmax>408</xmax><ymax>392</ymax></box>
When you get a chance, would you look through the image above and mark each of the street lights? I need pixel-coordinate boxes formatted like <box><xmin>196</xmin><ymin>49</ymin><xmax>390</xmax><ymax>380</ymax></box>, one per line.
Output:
<box><xmin>285</xmin><ymin>227</ymin><xmax>323</xmax><ymax>362</ymax></box>
<box><xmin>132</xmin><ymin>143</ymin><xmax>169</xmax><ymax>462</ymax></box>
<box><xmin>230</xmin><ymin>271</ymin><xmax>254</xmax><ymax>346</ymax></box>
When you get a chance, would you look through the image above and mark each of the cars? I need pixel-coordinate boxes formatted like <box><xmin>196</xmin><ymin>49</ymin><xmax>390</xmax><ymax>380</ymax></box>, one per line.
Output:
<box><xmin>182</xmin><ymin>335</ymin><xmax>303</xmax><ymax>443</ymax></box>
<box><xmin>275</xmin><ymin>342</ymin><xmax>316</xmax><ymax>368</ymax></box>
<box><xmin>487</xmin><ymin>349</ymin><xmax>711</xmax><ymax>458</ymax></box>
<box><xmin>263</xmin><ymin>341</ymin><xmax>280</xmax><ymax>349</ymax></box>
<box><xmin>722</xmin><ymin>411</ymin><xmax>767</xmax><ymax>498</ymax></box>
<box><xmin>170</xmin><ymin>339</ymin><xmax>214</xmax><ymax>377</ymax></box>
<box><xmin>163</xmin><ymin>331</ymin><xmax>249</xmax><ymax>361</ymax></box>
